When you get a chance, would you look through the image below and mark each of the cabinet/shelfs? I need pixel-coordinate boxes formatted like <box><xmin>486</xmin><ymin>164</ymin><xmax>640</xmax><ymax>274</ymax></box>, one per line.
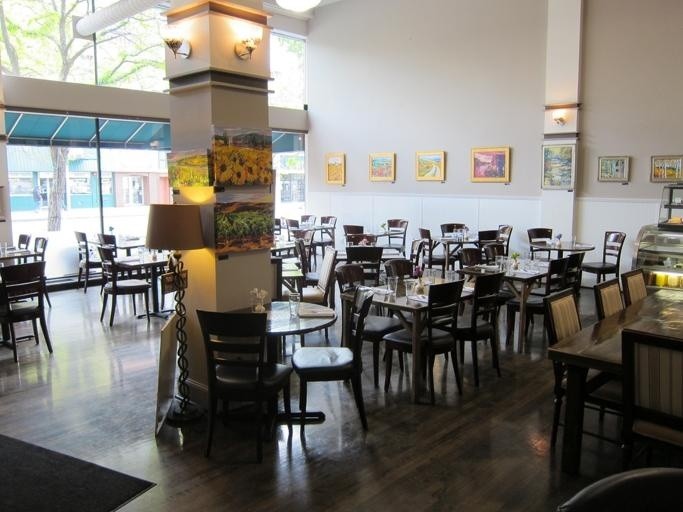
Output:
<box><xmin>656</xmin><ymin>183</ymin><xmax>683</xmax><ymax>231</ymax></box>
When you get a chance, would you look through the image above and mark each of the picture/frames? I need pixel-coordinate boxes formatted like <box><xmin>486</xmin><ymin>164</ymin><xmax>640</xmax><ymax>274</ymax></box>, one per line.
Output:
<box><xmin>471</xmin><ymin>146</ymin><xmax>511</xmax><ymax>183</ymax></box>
<box><xmin>541</xmin><ymin>143</ymin><xmax>577</xmax><ymax>192</ymax></box>
<box><xmin>324</xmin><ymin>152</ymin><xmax>345</xmax><ymax>186</ymax></box>
<box><xmin>369</xmin><ymin>151</ymin><xmax>395</xmax><ymax>182</ymax></box>
<box><xmin>416</xmin><ymin>149</ymin><xmax>445</xmax><ymax>181</ymax></box>
<box><xmin>597</xmin><ymin>155</ymin><xmax>630</xmax><ymax>183</ymax></box>
<box><xmin>650</xmin><ymin>154</ymin><xmax>683</xmax><ymax>183</ymax></box>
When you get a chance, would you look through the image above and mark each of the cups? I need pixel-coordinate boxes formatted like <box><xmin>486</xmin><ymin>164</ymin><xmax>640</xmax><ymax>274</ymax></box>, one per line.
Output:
<box><xmin>0</xmin><ymin>240</ymin><xmax>6</xmax><ymax>257</ymax></box>
<box><xmin>545</xmin><ymin>237</ymin><xmax>551</xmax><ymax>249</ymax></box>
<box><xmin>520</xmin><ymin>258</ymin><xmax>546</xmax><ymax>275</ymax></box>
<box><xmin>384</xmin><ymin>276</ymin><xmax>388</xmax><ymax>295</ymax></box>
<box><xmin>423</xmin><ymin>267</ymin><xmax>457</xmax><ymax>292</ymax></box>
<box><xmin>137</xmin><ymin>247</ymin><xmax>144</xmax><ymax>260</ymax></box>
<box><xmin>288</xmin><ymin>293</ymin><xmax>300</xmax><ymax>319</ymax></box>
<box><xmin>387</xmin><ymin>276</ymin><xmax>397</xmax><ymax>297</ymax></box>
<box><xmin>404</xmin><ymin>280</ymin><xmax>415</xmax><ymax>296</ymax></box>
<box><xmin>494</xmin><ymin>255</ymin><xmax>511</xmax><ymax>272</ymax></box>
<box><xmin>453</xmin><ymin>228</ymin><xmax>469</xmax><ymax>242</ymax></box>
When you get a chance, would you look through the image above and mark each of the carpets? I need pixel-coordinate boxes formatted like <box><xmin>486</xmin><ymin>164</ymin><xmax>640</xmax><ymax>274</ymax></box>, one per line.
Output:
<box><xmin>1</xmin><ymin>433</ymin><xmax>157</xmax><ymax>511</ymax></box>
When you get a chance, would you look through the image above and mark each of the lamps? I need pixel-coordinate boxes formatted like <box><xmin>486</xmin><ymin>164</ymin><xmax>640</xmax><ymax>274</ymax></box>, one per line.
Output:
<box><xmin>234</xmin><ymin>35</ymin><xmax>262</xmax><ymax>63</ymax></box>
<box><xmin>160</xmin><ymin>27</ymin><xmax>193</xmax><ymax>59</ymax></box>
<box><xmin>552</xmin><ymin>108</ymin><xmax>568</xmax><ymax>127</ymax></box>
<box><xmin>143</xmin><ymin>201</ymin><xmax>209</xmax><ymax>452</ymax></box>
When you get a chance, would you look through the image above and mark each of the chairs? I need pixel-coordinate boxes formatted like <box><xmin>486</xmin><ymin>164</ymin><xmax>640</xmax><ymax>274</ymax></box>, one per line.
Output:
<box><xmin>1</xmin><ymin>213</ymin><xmax>176</xmax><ymax>368</ymax></box>
<box><xmin>194</xmin><ymin>307</ymin><xmax>295</xmax><ymax>468</ymax></box>
